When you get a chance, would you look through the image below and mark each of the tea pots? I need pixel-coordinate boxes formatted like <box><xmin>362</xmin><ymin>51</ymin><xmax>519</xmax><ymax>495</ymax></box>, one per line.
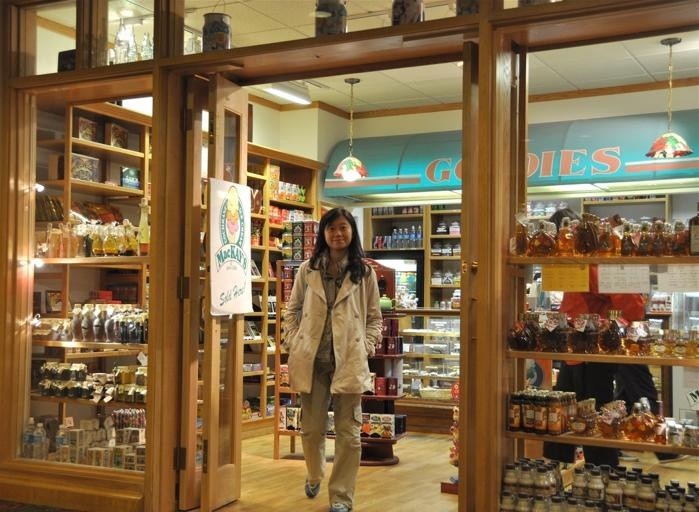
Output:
<box><xmin>379</xmin><ymin>295</ymin><xmax>397</xmax><ymax>312</ymax></box>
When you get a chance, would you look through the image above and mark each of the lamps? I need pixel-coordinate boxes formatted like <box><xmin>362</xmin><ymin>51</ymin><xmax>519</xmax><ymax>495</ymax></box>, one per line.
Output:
<box><xmin>331</xmin><ymin>75</ymin><xmax>369</xmax><ymax>184</ymax></box>
<box><xmin>644</xmin><ymin>35</ymin><xmax>694</xmax><ymax>159</ymax></box>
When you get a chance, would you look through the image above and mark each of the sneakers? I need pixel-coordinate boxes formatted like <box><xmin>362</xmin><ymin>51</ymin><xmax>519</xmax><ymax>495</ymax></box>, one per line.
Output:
<box><xmin>659</xmin><ymin>454</ymin><xmax>690</xmax><ymax>463</ymax></box>
<box><xmin>617</xmin><ymin>451</ymin><xmax>639</xmax><ymax>462</ymax></box>
<box><xmin>305</xmin><ymin>476</ymin><xmax>320</xmax><ymax>497</ymax></box>
<box><xmin>330</xmin><ymin>502</ymin><xmax>349</xmax><ymax>512</ymax></box>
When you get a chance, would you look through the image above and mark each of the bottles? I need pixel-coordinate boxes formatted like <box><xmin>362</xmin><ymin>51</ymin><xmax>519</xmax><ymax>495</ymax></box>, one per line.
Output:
<box><xmin>22</xmin><ymin>417</ymin><xmax>66</xmax><ymax>461</ymax></box>
<box><xmin>507</xmin><ymin>307</ymin><xmax>698</xmax><ymax>361</ymax></box>
<box><xmin>502</xmin><ymin>453</ymin><xmax>699</xmax><ymax>512</ymax></box>
<box><xmin>58</xmin><ymin>300</ymin><xmax>148</xmax><ymax>344</ymax></box>
<box><xmin>33</xmin><ymin>197</ymin><xmax>150</xmax><ymax>259</ymax></box>
<box><xmin>395</xmin><ymin>271</ymin><xmax>416</xmax><ymax>291</ymax></box>
<box><xmin>506</xmin><ymin>211</ymin><xmax>699</xmax><ymax>258</ymax></box>
<box><xmin>372</xmin><ymin>207</ymin><xmax>422</xmax><ymax>248</ymax></box>
<box><xmin>38</xmin><ymin>360</ymin><xmax>149</xmax><ymax>405</ymax></box>
<box><xmin>431</xmin><ymin>220</ymin><xmax>461</xmax><ymax>310</ymax></box>
<box><xmin>505</xmin><ymin>382</ymin><xmax>699</xmax><ymax>447</ymax></box>
<box><xmin>410</xmin><ymin>377</ymin><xmax>438</xmax><ymax>394</ymax></box>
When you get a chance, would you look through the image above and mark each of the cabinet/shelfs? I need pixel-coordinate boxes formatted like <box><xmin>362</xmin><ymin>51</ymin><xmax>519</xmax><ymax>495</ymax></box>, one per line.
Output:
<box><xmin>502</xmin><ymin>252</ymin><xmax>699</xmax><ymax>458</ymax></box>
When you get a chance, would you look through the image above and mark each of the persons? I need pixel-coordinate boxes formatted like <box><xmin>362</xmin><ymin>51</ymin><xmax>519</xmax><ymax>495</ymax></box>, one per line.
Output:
<box><xmin>541</xmin><ymin>207</ymin><xmax>648</xmax><ymax>470</ymax></box>
<box><xmin>618</xmin><ymin>363</ymin><xmax>690</xmax><ymax>465</ymax></box>
<box><xmin>284</xmin><ymin>206</ymin><xmax>384</xmax><ymax>512</ymax></box>
